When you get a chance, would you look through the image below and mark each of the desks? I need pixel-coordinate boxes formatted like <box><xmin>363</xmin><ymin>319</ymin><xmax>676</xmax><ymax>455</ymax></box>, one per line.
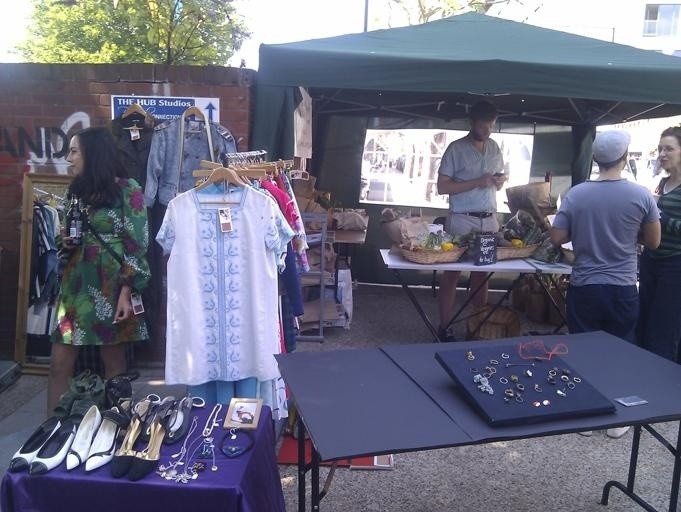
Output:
<box><xmin>273</xmin><ymin>330</ymin><xmax>681</xmax><ymax>512</ymax></box>
<box><xmin>0</xmin><ymin>404</ymin><xmax>284</xmax><ymax>511</ymax></box>
<box><xmin>328</xmin><ymin>215</ymin><xmax>369</xmax><ymax>330</ymax></box>
<box><xmin>379</xmin><ymin>248</ymin><xmax>538</xmax><ymax>343</ymax></box>
<box><xmin>524</xmin><ymin>256</ymin><xmax>639</xmax><ymax>336</ymax></box>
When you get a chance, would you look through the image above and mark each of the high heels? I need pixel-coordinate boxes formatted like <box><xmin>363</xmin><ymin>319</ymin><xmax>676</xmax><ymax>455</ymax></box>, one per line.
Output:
<box><xmin>65</xmin><ymin>405</ymin><xmax>172</xmax><ymax>482</ymax></box>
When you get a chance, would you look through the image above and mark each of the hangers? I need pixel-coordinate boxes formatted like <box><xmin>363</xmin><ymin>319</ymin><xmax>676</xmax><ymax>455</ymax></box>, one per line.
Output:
<box><xmin>32</xmin><ymin>188</ymin><xmax>64</xmax><ymax>208</ymax></box>
<box><xmin>184</xmin><ymin>96</ymin><xmax>205</xmax><ymax>133</ymax></box>
<box><xmin>120</xmin><ymin>91</ymin><xmax>146</xmax><ymax>130</ymax></box>
<box><xmin>189</xmin><ymin>149</ymin><xmax>294</xmax><ymax>206</ymax></box>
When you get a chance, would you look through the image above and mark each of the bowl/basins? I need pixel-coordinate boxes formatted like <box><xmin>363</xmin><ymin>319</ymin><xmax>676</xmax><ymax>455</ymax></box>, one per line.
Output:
<box><xmin>561</xmin><ymin>248</ymin><xmax>575</xmax><ymax>264</ymax></box>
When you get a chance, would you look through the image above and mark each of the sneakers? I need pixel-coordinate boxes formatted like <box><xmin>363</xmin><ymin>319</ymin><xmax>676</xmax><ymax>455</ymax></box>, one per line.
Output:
<box><xmin>433</xmin><ymin>323</ymin><xmax>457</xmax><ymax>342</ymax></box>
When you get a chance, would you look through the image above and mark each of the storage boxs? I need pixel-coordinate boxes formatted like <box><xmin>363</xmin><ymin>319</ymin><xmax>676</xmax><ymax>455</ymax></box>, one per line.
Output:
<box><xmin>292</xmin><ymin>175</ymin><xmax>332</xmax><ymax>229</ymax></box>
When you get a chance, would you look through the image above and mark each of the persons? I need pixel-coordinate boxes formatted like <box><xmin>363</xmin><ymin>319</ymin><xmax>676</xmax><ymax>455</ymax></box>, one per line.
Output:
<box><xmin>629</xmin><ymin>148</ymin><xmax>663</xmax><ymax>182</ymax></box>
<box><xmin>45</xmin><ymin>124</ymin><xmax>153</xmax><ymax>422</ymax></box>
<box><xmin>434</xmin><ymin>99</ymin><xmax>508</xmax><ymax>342</ymax></box>
<box><xmin>549</xmin><ymin>127</ymin><xmax>663</xmax><ymax>440</ymax></box>
<box><xmin>635</xmin><ymin>125</ymin><xmax>681</xmax><ymax>364</ymax></box>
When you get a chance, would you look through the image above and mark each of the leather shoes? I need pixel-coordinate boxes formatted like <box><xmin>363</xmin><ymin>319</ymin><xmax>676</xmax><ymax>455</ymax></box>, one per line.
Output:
<box><xmin>7</xmin><ymin>416</ymin><xmax>77</xmax><ymax>475</ymax></box>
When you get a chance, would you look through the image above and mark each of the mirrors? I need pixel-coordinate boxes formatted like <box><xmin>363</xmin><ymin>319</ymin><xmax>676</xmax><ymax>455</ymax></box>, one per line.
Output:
<box><xmin>14</xmin><ymin>172</ymin><xmax>75</xmax><ymax>375</ymax></box>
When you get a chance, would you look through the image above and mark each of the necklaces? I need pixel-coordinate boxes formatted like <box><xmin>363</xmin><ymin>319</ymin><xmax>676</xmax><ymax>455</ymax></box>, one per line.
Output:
<box><xmin>663</xmin><ymin>178</ymin><xmax>679</xmax><ymax>196</ymax></box>
<box><xmin>157</xmin><ymin>402</ymin><xmax>226</xmax><ymax>484</ymax></box>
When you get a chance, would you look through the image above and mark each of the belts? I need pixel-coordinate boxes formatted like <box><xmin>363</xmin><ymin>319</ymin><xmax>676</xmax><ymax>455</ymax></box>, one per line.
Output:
<box><xmin>458</xmin><ymin>210</ymin><xmax>494</xmax><ymax>219</ymax></box>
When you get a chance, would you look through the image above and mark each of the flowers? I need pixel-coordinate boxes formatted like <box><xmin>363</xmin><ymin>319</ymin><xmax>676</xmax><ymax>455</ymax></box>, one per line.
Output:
<box><xmin>511</xmin><ymin>238</ymin><xmax>525</xmax><ymax>248</ymax></box>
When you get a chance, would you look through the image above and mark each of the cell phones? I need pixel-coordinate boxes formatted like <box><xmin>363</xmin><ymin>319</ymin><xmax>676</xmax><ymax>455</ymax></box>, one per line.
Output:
<box><xmin>493</xmin><ymin>170</ymin><xmax>505</xmax><ymax>177</ymax></box>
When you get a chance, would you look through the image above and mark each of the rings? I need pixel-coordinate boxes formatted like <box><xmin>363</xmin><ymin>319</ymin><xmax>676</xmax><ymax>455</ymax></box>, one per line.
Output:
<box><xmin>465</xmin><ymin>350</ymin><xmax>584</xmax><ymax>407</ymax></box>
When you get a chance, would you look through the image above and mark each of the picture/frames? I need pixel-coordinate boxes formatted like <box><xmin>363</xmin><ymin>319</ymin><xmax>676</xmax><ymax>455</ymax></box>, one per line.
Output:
<box><xmin>223</xmin><ymin>397</ymin><xmax>263</xmax><ymax>430</ymax></box>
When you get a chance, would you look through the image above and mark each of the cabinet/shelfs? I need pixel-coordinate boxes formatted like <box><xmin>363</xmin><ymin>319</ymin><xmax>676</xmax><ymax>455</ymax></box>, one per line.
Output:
<box><xmin>293</xmin><ymin>212</ymin><xmax>327</xmax><ymax>343</ymax></box>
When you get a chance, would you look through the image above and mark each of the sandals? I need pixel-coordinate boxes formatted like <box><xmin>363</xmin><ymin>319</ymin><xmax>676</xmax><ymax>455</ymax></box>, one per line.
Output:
<box><xmin>52</xmin><ymin>370</ymin><xmax>194</xmax><ymax>446</ymax></box>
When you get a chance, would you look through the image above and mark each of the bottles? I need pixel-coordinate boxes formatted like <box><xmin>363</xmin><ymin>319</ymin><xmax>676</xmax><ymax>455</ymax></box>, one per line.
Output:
<box><xmin>64</xmin><ymin>186</ymin><xmax>83</xmax><ymax>248</ymax></box>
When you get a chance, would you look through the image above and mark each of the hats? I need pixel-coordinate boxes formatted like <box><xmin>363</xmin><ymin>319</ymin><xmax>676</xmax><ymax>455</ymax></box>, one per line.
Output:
<box><xmin>591</xmin><ymin>129</ymin><xmax>631</xmax><ymax>163</ymax></box>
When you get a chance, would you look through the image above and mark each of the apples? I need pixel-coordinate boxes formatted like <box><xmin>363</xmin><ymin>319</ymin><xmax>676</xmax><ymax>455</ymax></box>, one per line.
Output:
<box><xmin>512</xmin><ymin>239</ymin><xmax>523</xmax><ymax>247</ymax></box>
